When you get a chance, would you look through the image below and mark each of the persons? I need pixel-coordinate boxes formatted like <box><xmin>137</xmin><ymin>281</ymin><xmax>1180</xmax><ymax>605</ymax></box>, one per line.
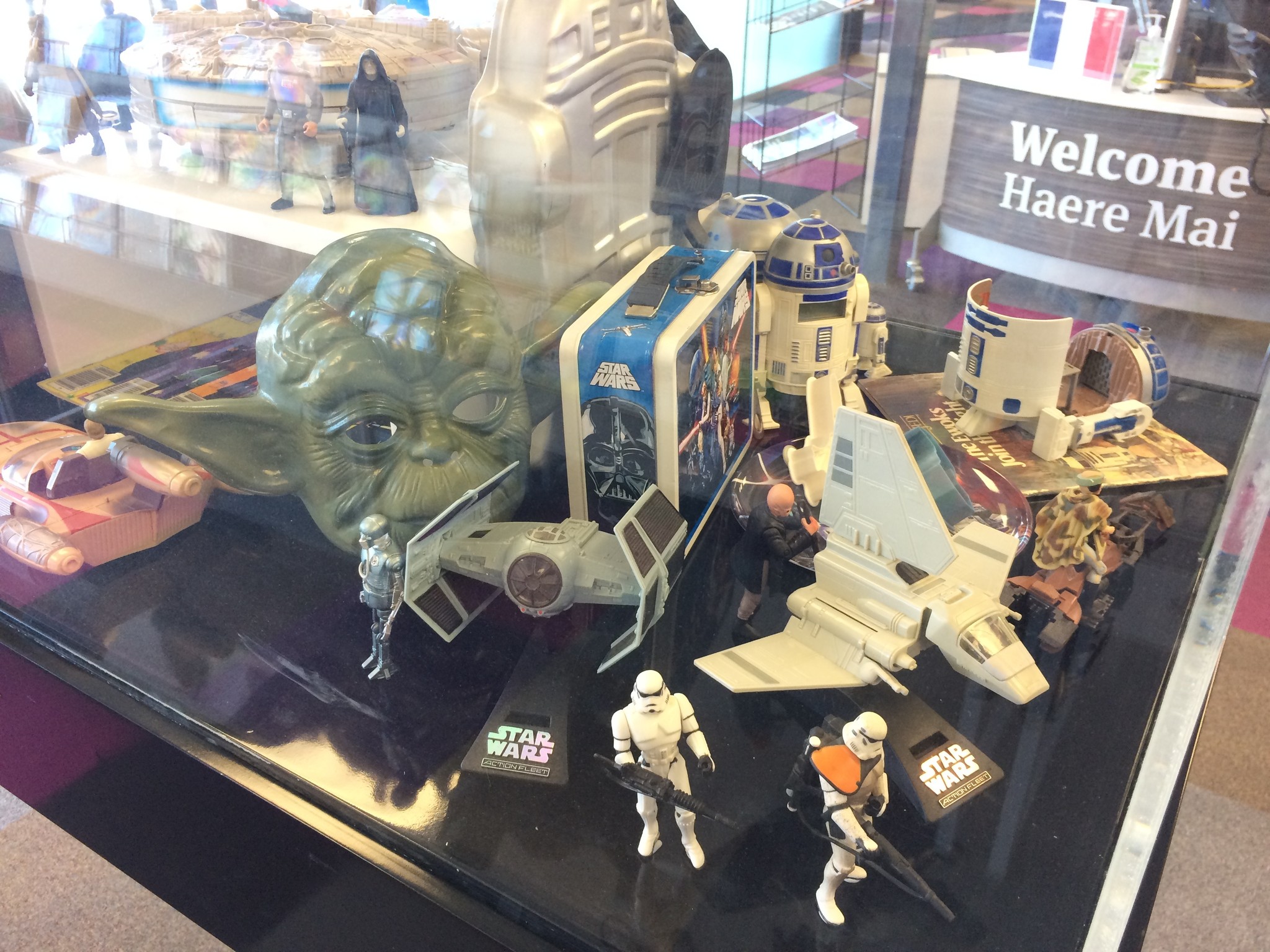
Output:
<box><xmin>256</xmin><ymin>40</ymin><xmax>336</xmax><ymax>215</ymax></box>
<box><xmin>730</xmin><ymin>483</ymin><xmax>819</xmax><ymax>640</ymax></box>
<box><xmin>611</xmin><ymin>670</ymin><xmax>715</xmax><ymax>871</ymax></box>
<box><xmin>23</xmin><ymin>13</ymin><xmax>107</xmax><ymax>157</ymax></box>
<box><xmin>1030</xmin><ymin>469</ymin><xmax>1113</xmax><ymax>623</ymax></box>
<box><xmin>814</xmin><ymin>712</ymin><xmax>891</xmax><ymax>928</ymax></box>
<box><xmin>335</xmin><ymin>48</ymin><xmax>421</xmax><ymax>219</ymax></box>
<box><xmin>77</xmin><ymin>0</ymin><xmax>145</xmax><ymax>133</ymax></box>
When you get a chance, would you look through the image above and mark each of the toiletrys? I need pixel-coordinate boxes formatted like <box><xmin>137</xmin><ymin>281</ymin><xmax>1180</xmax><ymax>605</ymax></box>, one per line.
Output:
<box><xmin>1121</xmin><ymin>7</ymin><xmax>1171</xmax><ymax>103</ymax></box>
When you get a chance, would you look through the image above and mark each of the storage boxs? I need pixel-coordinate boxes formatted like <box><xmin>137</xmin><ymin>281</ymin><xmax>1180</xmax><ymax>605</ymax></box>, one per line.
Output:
<box><xmin>561</xmin><ymin>246</ymin><xmax>758</xmax><ymax>559</ymax></box>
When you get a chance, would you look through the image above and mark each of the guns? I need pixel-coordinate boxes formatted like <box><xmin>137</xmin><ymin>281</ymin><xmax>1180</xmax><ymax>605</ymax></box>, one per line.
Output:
<box><xmin>593</xmin><ymin>753</ymin><xmax>740</xmax><ymax>830</ymax></box>
<box><xmin>788</xmin><ymin>771</ymin><xmax>955</xmax><ymax>924</ymax></box>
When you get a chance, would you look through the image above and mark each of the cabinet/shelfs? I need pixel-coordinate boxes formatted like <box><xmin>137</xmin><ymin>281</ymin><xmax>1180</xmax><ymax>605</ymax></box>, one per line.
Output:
<box><xmin>737</xmin><ymin>0</ymin><xmax>888</xmax><ymax>219</ymax></box>
